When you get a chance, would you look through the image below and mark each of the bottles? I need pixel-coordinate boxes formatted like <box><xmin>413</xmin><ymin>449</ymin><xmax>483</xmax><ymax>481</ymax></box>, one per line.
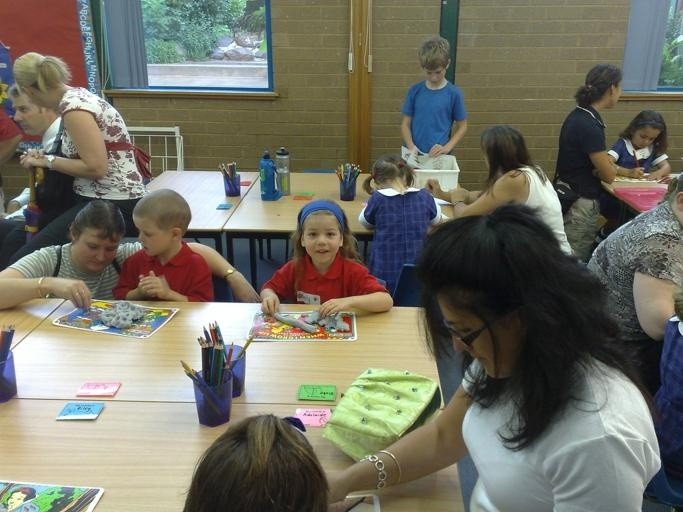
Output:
<box><xmin>259</xmin><ymin>151</ymin><xmax>276</xmax><ymax>199</ymax></box>
<box><xmin>274</xmin><ymin>146</ymin><xmax>291</xmax><ymax>196</ymax></box>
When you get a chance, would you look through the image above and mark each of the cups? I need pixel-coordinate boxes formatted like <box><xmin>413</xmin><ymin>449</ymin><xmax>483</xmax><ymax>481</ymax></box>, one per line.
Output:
<box><xmin>220</xmin><ymin>344</ymin><xmax>246</xmax><ymax>398</ymax></box>
<box><xmin>192</xmin><ymin>369</ymin><xmax>232</xmax><ymax>427</ymax></box>
<box><xmin>0</xmin><ymin>350</ymin><xmax>18</xmax><ymax>403</ymax></box>
<box><xmin>340</xmin><ymin>181</ymin><xmax>356</xmax><ymax>202</ymax></box>
<box><xmin>222</xmin><ymin>173</ymin><xmax>241</xmax><ymax>196</ymax></box>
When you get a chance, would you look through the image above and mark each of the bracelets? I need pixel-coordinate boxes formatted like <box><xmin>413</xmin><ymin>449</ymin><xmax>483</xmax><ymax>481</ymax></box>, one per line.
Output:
<box><xmin>379</xmin><ymin>451</ymin><xmax>402</xmax><ymax>487</ymax></box>
<box><xmin>359</xmin><ymin>454</ymin><xmax>388</xmax><ymax>491</ymax></box>
<box><xmin>615</xmin><ymin>165</ymin><xmax>619</xmax><ymax>175</ymax></box>
<box><xmin>37</xmin><ymin>275</ymin><xmax>49</xmax><ymax>298</ymax></box>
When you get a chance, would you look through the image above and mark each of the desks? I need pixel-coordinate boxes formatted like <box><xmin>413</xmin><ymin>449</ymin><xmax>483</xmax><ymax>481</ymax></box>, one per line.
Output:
<box><xmin>4</xmin><ymin>295</ymin><xmax>466</xmax><ymax>508</ymax></box>
<box><xmin>132</xmin><ymin>167</ymin><xmax>471</xmax><ymax>302</ymax></box>
<box><xmin>600</xmin><ymin>167</ymin><xmax>683</xmax><ymax>226</ymax></box>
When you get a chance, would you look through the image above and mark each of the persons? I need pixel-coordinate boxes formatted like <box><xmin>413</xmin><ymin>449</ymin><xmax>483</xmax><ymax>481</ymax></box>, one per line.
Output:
<box><xmin>180</xmin><ymin>414</ymin><xmax>332</xmax><ymax>512</ymax></box>
<box><xmin>357</xmin><ymin>152</ymin><xmax>443</xmax><ymax>296</ymax></box>
<box><xmin>322</xmin><ymin>202</ymin><xmax>662</xmax><ymax>512</ymax></box>
<box><xmin>0</xmin><ymin>51</ymin><xmax>151</xmax><ymax>271</ymax></box>
<box><xmin>111</xmin><ymin>188</ymin><xmax>213</xmax><ymax>302</ymax></box>
<box><xmin>402</xmin><ymin>36</ymin><xmax>467</xmax><ymax>159</ymax></box>
<box><xmin>590</xmin><ymin>111</ymin><xmax>671</xmax><ymax>255</ymax></box>
<box><xmin>425</xmin><ymin>125</ymin><xmax>574</xmax><ymax>255</ymax></box>
<box><xmin>259</xmin><ymin>200</ymin><xmax>393</xmax><ymax>316</ymax></box>
<box><xmin>553</xmin><ymin>64</ymin><xmax>622</xmax><ymax>263</ymax></box>
<box><xmin>641</xmin><ymin>292</ymin><xmax>683</xmax><ymax>510</ymax></box>
<box><xmin>586</xmin><ymin>171</ymin><xmax>683</xmax><ymax>397</ymax></box>
<box><xmin>1</xmin><ymin>199</ymin><xmax>262</xmax><ymax>311</ymax></box>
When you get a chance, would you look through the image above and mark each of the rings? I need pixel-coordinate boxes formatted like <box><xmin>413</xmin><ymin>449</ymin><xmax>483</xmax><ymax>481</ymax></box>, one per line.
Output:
<box><xmin>74</xmin><ymin>292</ymin><xmax>79</xmax><ymax>297</ymax></box>
<box><xmin>80</xmin><ymin>290</ymin><xmax>85</xmax><ymax>295</ymax></box>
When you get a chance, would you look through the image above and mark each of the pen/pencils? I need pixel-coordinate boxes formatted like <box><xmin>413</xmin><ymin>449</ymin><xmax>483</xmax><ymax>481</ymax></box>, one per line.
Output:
<box><xmin>633</xmin><ymin>151</ymin><xmax>639</xmax><ymax>167</ymax></box>
<box><xmin>0</xmin><ymin>323</ymin><xmax>16</xmax><ymax>397</ymax></box>
<box><xmin>218</xmin><ymin>160</ymin><xmax>238</xmax><ymax>194</ymax></box>
<box><xmin>335</xmin><ymin>160</ymin><xmax>362</xmax><ymax>201</ymax></box>
<box><xmin>180</xmin><ymin>321</ymin><xmax>254</xmax><ymax>421</ymax></box>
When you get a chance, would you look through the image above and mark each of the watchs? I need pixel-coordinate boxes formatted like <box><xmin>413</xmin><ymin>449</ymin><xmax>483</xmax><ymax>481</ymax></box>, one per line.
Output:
<box><xmin>223</xmin><ymin>267</ymin><xmax>237</xmax><ymax>280</ymax></box>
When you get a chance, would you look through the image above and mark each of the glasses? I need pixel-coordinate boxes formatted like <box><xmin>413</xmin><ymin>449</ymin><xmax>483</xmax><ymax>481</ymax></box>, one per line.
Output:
<box><xmin>438</xmin><ymin>312</ymin><xmax>505</xmax><ymax>349</ymax></box>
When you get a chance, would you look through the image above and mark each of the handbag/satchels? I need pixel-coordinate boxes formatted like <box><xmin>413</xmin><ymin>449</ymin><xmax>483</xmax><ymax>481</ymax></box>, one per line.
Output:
<box><xmin>34</xmin><ymin>129</ymin><xmax>76</xmax><ymax>214</ymax></box>
<box><xmin>322</xmin><ymin>366</ymin><xmax>442</xmax><ymax>465</ymax></box>
<box><xmin>552</xmin><ymin>184</ymin><xmax>578</xmax><ymax>217</ymax></box>
